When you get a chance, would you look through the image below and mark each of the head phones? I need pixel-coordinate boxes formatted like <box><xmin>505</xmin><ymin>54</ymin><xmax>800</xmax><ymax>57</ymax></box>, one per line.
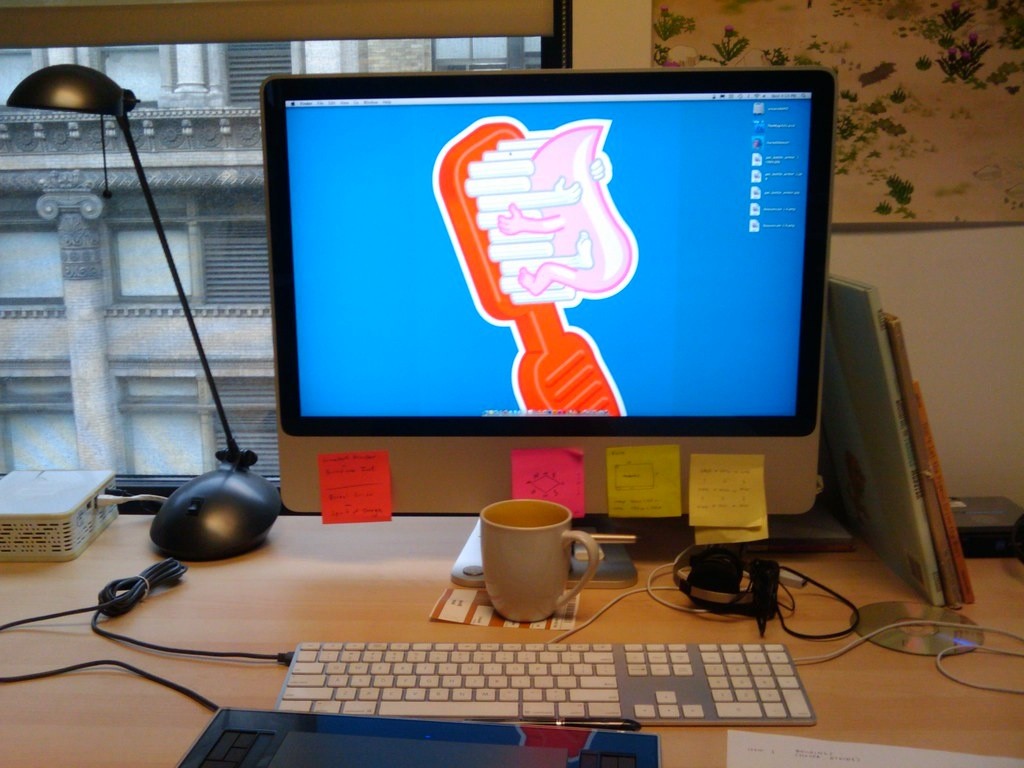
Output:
<box><xmin>674</xmin><ymin>546</ymin><xmax>779</xmax><ymax>622</ymax></box>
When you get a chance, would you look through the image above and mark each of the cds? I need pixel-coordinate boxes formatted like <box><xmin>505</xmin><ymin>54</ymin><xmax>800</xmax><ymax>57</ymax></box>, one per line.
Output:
<box><xmin>850</xmin><ymin>601</ymin><xmax>984</xmax><ymax>656</ymax></box>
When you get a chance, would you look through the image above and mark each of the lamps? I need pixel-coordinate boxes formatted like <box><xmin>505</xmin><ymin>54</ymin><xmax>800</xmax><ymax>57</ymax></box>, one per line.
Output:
<box><xmin>6</xmin><ymin>65</ymin><xmax>283</xmax><ymax>564</ymax></box>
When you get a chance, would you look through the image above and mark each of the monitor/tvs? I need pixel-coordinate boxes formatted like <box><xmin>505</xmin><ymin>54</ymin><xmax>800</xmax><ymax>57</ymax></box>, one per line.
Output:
<box><xmin>261</xmin><ymin>65</ymin><xmax>838</xmax><ymax>587</ymax></box>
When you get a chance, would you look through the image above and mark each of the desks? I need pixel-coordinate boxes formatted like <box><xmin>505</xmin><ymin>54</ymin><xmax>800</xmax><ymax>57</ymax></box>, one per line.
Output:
<box><xmin>0</xmin><ymin>516</ymin><xmax>1024</xmax><ymax>768</ymax></box>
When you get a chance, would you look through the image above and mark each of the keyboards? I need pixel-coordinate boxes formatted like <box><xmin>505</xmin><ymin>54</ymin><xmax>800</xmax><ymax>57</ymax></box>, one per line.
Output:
<box><xmin>274</xmin><ymin>643</ymin><xmax>818</xmax><ymax>728</ymax></box>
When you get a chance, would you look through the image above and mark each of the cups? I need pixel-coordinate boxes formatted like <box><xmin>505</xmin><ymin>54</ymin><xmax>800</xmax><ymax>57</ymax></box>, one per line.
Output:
<box><xmin>480</xmin><ymin>500</ymin><xmax>598</xmax><ymax>622</ymax></box>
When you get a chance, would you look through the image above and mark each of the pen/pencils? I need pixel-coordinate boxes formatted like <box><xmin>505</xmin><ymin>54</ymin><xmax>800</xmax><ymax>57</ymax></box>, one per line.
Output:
<box><xmin>528</xmin><ymin>717</ymin><xmax>641</xmax><ymax>731</ymax></box>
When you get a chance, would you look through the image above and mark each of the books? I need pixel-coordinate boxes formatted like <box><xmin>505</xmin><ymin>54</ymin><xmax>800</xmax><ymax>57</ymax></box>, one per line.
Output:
<box><xmin>827</xmin><ymin>271</ymin><xmax>944</xmax><ymax>607</ymax></box>
<box><xmin>912</xmin><ymin>379</ymin><xmax>975</xmax><ymax>604</ymax></box>
<box><xmin>883</xmin><ymin>311</ymin><xmax>961</xmax><ymax>607</ymax></box>
